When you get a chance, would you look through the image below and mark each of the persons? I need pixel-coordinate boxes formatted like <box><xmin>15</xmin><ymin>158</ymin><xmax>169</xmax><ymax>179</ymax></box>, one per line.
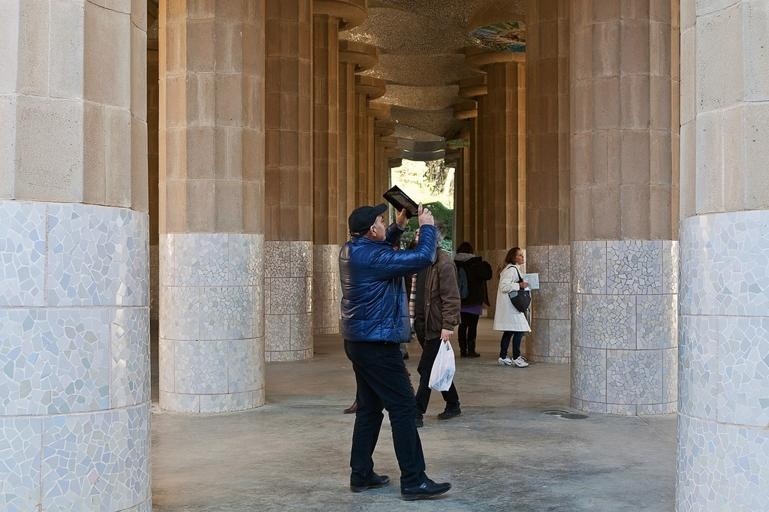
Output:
<box><xmin>452</xmin><ymin>242</ymin><xmax>493</xmax><ymax>358</ymax></box>
<box><xmin>409</xmin><ymin>219</ymin><xmax>463</xmax><ymax>429</ymax></box>
<box><xmin>343</xmin><ymin>343</ymin><xmax>406</xmax><ymax>414</ymax></box>
<box><xmin>339</xmin><ymin>202</ymin><xmax>451</xmax><ymax>500</ymax></box>
<box><xmin>493</xmin><ymin>247</ymin><xmax>531</xmax><ymax>367</ymax></box>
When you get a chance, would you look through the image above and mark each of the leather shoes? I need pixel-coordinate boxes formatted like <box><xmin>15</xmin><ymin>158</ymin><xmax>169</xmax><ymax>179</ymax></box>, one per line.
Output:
<box><xmin>343</xmin><ymin>401</ymin><xmax>357</xmax><ymax>414</ymax></box>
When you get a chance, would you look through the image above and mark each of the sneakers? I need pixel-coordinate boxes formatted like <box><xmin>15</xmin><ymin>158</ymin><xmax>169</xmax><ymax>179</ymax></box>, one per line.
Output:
<box><xmin>511</xmin><ymin>355</ymin><xmax>529</xmax><ymax>368</ymax></box>
<box><xmin>497</xmin><ymin>355</ymin><xmax>512</xmax><ymax>365</ymax></box>
<box><xmin>401</xmin><ymin>479</ymin><xmax>452</xmax><ymax>500</ymax></box>
<box><xmin>460</xmin><ymin>350</ymin><xmax>480</xmax><ymax>358</ymax></box>
<box><xmin>439</xmin><ymin>408</ymin><xmax>461</xmax><ymax>419</ymax></box>
<box><xmin>350</xmin><ymin>470</ymin><xmax>390</xmax><ymax>492</ymax></box>
<box><xmin>414</xmin><ymin>413</ymin><xmax>423</xmax><ymax>427</ymax></box>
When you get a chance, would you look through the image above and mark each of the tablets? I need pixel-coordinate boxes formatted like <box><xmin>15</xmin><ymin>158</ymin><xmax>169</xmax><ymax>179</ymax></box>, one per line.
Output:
<box><xmin>389</xmin><ymin>191</ymin><xmax>418</xmax><ymax>216</ymax></box>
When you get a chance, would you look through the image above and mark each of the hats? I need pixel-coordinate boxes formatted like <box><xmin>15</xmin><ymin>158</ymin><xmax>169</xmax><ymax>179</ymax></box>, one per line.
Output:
<box><xmin>348</xmin><ymin>203</ymin><xmax>387</xmax><ymax>237</ymax></box>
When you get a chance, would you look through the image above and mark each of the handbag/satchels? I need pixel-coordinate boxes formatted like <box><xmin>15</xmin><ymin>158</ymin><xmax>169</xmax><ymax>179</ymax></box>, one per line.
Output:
<box><xmin>508</xmin><ymin>279</ymin><xmax>531</xmax><ymax>312</ymax></box>
